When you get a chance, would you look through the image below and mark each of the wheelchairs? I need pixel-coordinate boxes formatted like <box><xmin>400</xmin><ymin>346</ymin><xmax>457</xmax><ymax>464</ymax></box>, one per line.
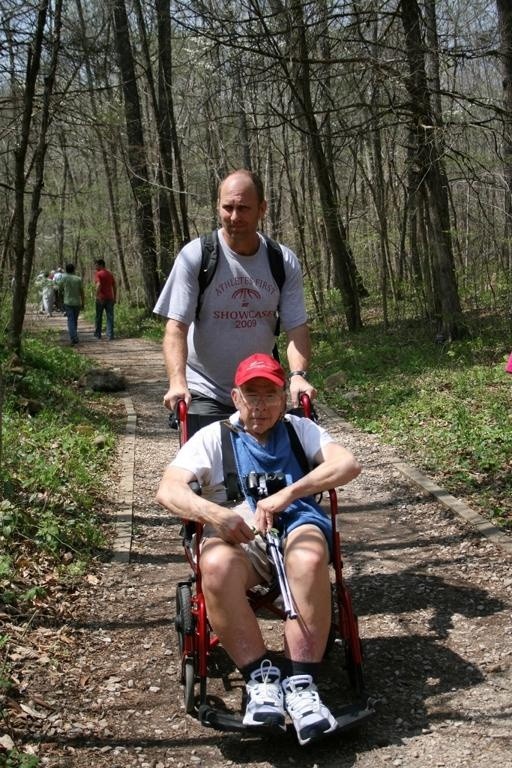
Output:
<box><xmin>167</xmin><ymin>390</ymin><xmax>389</xmax><ymax>741</ymax></box>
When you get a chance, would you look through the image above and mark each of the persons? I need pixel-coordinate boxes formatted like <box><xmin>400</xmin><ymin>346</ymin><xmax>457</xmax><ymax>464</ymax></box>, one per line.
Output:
<box><xmin>34</xmin><ymin>264</ymin><xmax>84</xmax><ymax>317</ymax></box>
<box><xmin>151</xmin><ymin>167</ymin><xmax>319</xmax><ymax>546</ymax></box>
<box><xmin>94</xmin><ymin>259</ymin><xmax>117</xmax><ymax>341</ymax></box>
<box><xmin>58</xmin><ymin>263</ymin><xmax>85</xmax><ymax>347</ymax></box>
<box><xmin>155</xmin><ymin>352</ymin><xmax>364</xmax><ymax>746</ymax></box>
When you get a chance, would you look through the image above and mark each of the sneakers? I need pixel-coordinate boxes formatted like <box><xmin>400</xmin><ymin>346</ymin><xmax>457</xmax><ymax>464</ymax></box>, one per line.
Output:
<box><xmin>282</xmin><ymin>674</ymin><xmax>339</xmax><ymax>744</ymax></box>
<box><xmin>243</xmin><ymin>666</ymin><xmax>286</xmax><ymax>725</ymax></box>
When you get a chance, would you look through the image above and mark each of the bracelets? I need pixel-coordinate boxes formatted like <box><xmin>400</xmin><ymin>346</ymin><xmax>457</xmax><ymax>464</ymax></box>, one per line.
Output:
<box><xmin>287</xmin><ymin>371</ymin><xmax>311</xmax><ymax>381</ymax></box>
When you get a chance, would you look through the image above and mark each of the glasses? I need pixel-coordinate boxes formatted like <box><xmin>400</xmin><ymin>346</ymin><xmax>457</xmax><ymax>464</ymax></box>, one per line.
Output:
<box><xmin>239</xmin><ymin>386</ymin><xmax>284</xmax><ymax>410</ymax></box>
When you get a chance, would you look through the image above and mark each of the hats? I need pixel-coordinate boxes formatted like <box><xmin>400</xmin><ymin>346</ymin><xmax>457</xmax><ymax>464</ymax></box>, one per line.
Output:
<box><xmin>235</xmin><ymin>354</ymin><xmax>285</xmax><ymax>387</ymax></box>
